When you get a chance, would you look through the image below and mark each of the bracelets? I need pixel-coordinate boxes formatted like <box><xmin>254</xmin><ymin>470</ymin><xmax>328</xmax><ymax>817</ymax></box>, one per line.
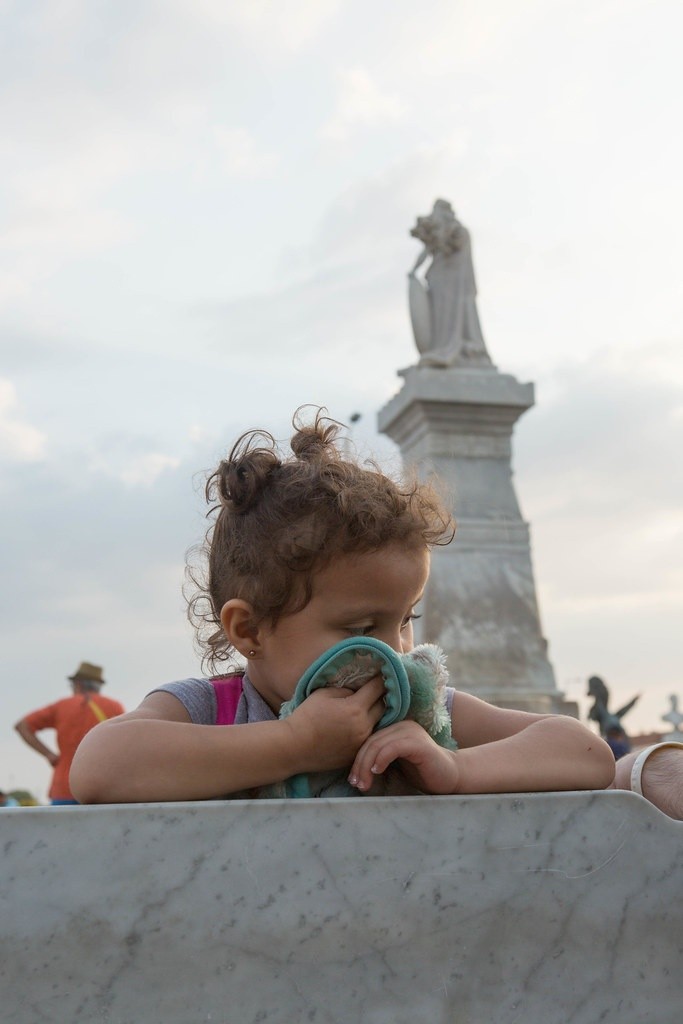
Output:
<box><xmin>631</xmin><ymin>742</ymin><xmax>683</xmax><ymax>797</ymax></box>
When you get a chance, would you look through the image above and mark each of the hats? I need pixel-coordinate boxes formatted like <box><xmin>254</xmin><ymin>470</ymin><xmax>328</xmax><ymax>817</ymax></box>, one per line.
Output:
<box><xmin>67</xmin><ymin>662</ymin><xmax>105</xmax><ymax>685</ymax></box>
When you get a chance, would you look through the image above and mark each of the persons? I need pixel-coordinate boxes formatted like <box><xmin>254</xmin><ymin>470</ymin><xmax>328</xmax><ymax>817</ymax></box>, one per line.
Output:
<box><xmin>68</xmin><ymin>405</ymin><xmax>617</xmax><ymax>804</ymax></box>
<box><xmin>397</xmin><ymin>200</ymin><xmax>494</xmax><ymax>372</ymax></box>
<box><xmin>608</xmin><ymin>742</ymin><xmax>683</xmax><ymax>821</ymax></box>
<box><xmin>1</xmin><ymin>662</ymin><xmax>125</xmax><ymax>807</ymax></box>
